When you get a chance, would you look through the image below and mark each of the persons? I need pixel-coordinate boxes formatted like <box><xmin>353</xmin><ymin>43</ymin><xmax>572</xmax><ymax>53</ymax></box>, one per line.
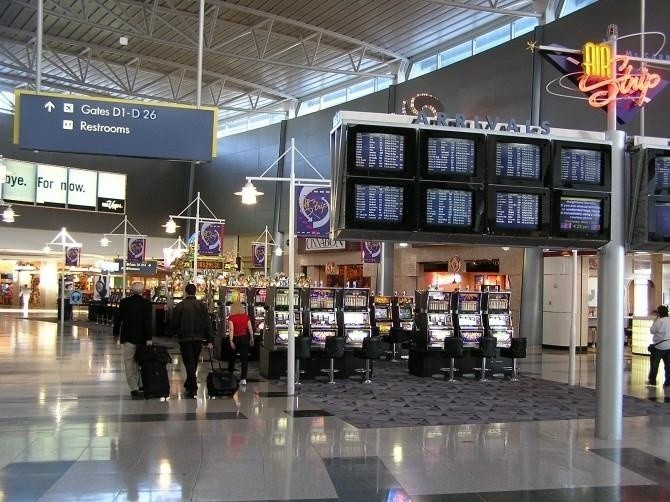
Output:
<box><xmin>228</xmin><ymin>302</ymin><xmax>254</xmax><ymax>385</ymax></box>
<box><xmin>644</xmin><ymin>304</ymin><xmax>670</xmax><ymax>386</ymax></box>
<box><xmin>18</xmin><ymin>284</ymin><xmax>32</xmax><ymax>310</ymax></box>
<box><xmin>166</xmin><ymin>283</ymin><xmax>213</xmax><ymax>396</ymax></box>
<box><xmin>113</xmin><ymin>282</ymin><xmax>152</xmax><ymax>394</ymax></box>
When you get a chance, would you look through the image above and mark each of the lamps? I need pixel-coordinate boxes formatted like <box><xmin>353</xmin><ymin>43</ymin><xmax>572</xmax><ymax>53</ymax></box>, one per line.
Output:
<box><xmin>0</xmin><ymin>179</ymin><xmax>285</xmax><ymax>257</ymax></box>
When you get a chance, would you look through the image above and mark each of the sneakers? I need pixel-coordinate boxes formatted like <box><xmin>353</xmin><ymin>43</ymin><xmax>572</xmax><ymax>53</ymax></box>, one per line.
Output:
<box><xmin>240</xmin><ymin>379</ymin><xmax>246</xmax><ymax>385</ymax></box>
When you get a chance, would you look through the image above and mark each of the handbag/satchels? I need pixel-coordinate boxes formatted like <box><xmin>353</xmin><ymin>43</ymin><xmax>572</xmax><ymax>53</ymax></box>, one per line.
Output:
<box><xmin>137</xmin><ymin>345</ymin><xmax>173</xmax><ymax>367</ymax></box>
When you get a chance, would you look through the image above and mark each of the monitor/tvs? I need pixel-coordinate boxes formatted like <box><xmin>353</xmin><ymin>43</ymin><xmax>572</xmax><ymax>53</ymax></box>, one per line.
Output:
<box><xmin>648</xmin><ymin>148</ymin><xmax>670</xmax><ymax>242</ymax></box>
<box><xmin>253</xmin><ymin>307</ymin><xmax>511</xmax><ymax>346</ymax></box>
<box><xmin>347</xmin><ymin>124</ymin><xmax>611</xmax><ymax>240</ymax></box>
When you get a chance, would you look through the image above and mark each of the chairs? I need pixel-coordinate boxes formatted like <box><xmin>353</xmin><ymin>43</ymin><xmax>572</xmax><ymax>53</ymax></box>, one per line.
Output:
<box><xmin>319</xmin><ymin>336</ymin><xmax>344</xmax><ymax>383</ymax></box>
<box><xmin>387</xmin><ymin>326</ymin><xmax>403</xmax><ymax>363</ymax></box>
<box><xmin>500</xmin><ymin>338</ymin><xmax>527</xmax><ymax>382</ymax></box>
<box><xmin>285</xmin><ymin>338</ymin><xmax>310</xmax><ymax>385</ymax></box>
<box><xmin>471</xmin><ymin>336</ymin><xmax>497</xmax><ymax>382</ymax></box>
<box><xmin>354</xmin><ymin>337</ymin><xmax>379</xmax><ymax>383</ymax></box>
<box><xmin>441</xmin><ymin>338</ymin><xmax>463</xmax><ymax>382</ymax></box>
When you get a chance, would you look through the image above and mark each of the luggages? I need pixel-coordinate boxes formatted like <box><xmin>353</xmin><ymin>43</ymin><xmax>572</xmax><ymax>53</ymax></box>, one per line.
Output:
<box><xmin>205</xmin><ymin>345</ymin><xmax>239</xmax><ymax>399</ymax></box>
<box><xmin>140</xmin><ymin>342</ymin><xmax>170</xmax><ymax>401</ymax></box>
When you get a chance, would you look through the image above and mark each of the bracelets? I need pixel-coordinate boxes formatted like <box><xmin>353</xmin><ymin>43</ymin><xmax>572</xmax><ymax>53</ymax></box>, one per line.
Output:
<box><xmin>229</xmin><ymin>339</ymin><xmax>233</xmax><ymax>341</ymax></box>
